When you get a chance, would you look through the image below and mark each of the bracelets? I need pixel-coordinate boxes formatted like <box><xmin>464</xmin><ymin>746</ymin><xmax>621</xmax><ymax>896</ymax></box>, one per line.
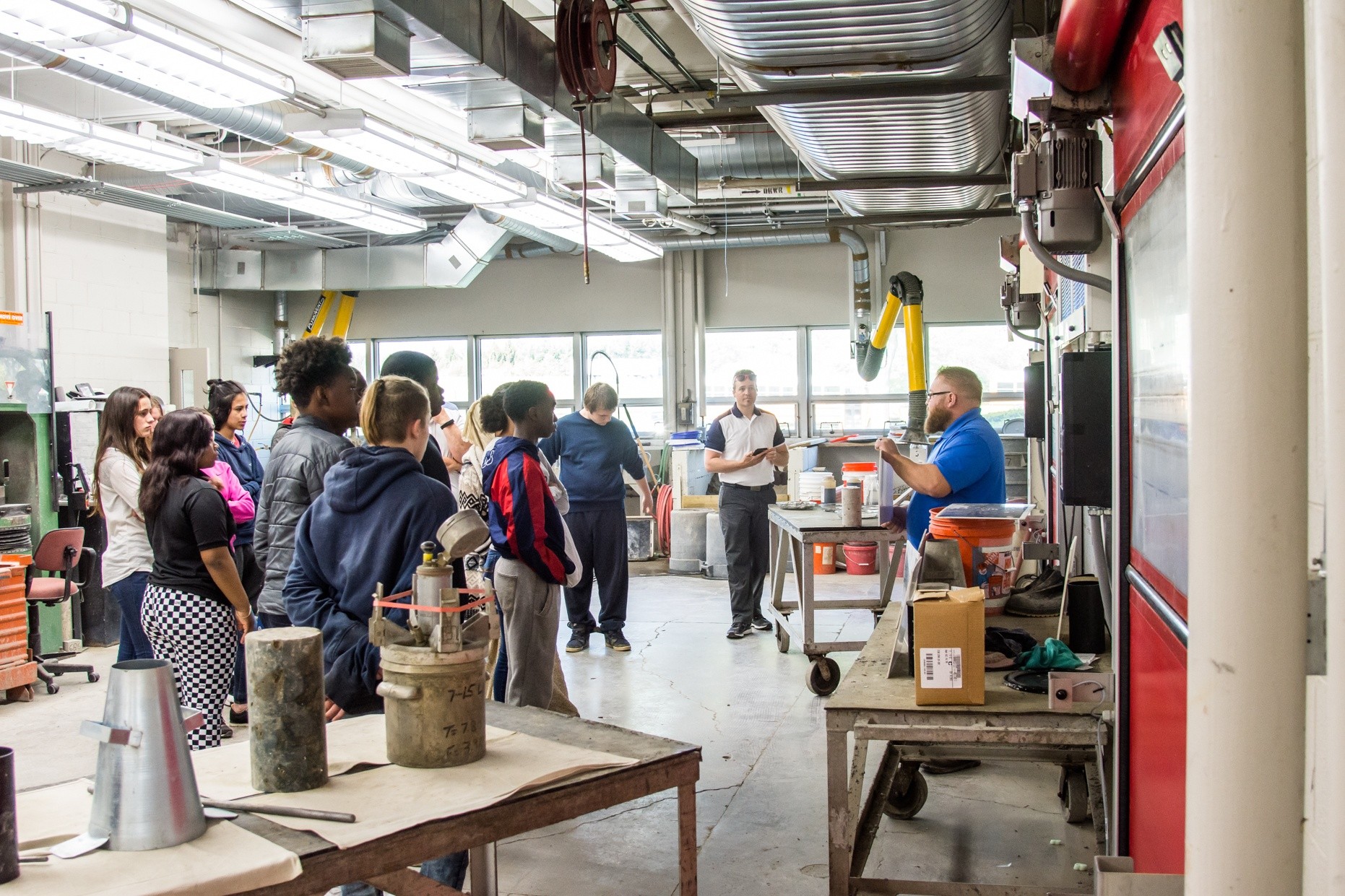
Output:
<box><xmin>236</xmin><ymin>604</ymin><xmax>252</xmax><ymax>618</ymax></box>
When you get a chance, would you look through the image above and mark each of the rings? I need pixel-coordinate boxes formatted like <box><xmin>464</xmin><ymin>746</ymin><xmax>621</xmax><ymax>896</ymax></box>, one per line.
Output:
<box><xmin>879</xmin><ymin>439</ymin><xmax>882</xmax><ymax>441</ymax></box>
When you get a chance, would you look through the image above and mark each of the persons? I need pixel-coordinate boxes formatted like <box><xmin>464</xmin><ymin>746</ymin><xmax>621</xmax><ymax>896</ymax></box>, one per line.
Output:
<box><xmin>704</xmin><ymin>370</ymin><xmax>790</xmax><ymax>639</ymax></box>
<box><xmin>537</xmin><ymin>382</ymin><xmax>653</xmax><ymax>652</ymax></box>
<box><xmin>91</xmin><ymin>336</ymin><xmax>583</xmax><ymax>896</ymax></box>
<box><xmin>874</xmin><ymin>366</ymin><xmax>1007</xmax><ymax>772</ymax></box>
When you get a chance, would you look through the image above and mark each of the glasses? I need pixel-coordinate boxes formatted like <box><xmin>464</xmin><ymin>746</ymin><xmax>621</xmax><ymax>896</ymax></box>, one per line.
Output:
<box><xmin>735</xmin><ymin>373</ymin><xmax>757</xmax><ymax>382</ymax></box>
<box><xmin>926</xmin><ymin>389</ymin><xmax>952</xmax><ymax>400</ymax></box>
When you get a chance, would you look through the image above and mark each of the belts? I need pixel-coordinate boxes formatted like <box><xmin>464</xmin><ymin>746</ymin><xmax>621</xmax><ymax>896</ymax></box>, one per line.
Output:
<box><xmin>721</xmin><ymin>482</ymin><xmax>774</xmax><ymax>492</ymax></box>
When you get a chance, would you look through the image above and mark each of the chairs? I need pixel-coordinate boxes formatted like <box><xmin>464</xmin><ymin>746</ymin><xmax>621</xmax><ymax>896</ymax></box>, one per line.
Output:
<box><xmin>28</xmin><ymin>526</ymin><xmax>100</xmax><ymax>695</ymax></box>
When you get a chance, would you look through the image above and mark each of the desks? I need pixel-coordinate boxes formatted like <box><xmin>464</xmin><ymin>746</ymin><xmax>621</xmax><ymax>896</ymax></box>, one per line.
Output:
<box><xmin>0</xmin><ymin>695</ymin><xmax>706</xmax><ymax>896</ymax></box>
<box><xmin>760</xmin><ymin>503</ymin><xmax>905</xmax><ymax>696</ymax></box>
<box><xmin>820</xmin><ymin>597</ymin><xmax>1109</xmax><ymax>896</ymax></box>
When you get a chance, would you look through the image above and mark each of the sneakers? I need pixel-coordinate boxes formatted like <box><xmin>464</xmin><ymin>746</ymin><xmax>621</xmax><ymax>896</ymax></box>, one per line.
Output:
<box><xmin>565</xmin><ymin>630</ymin><xmax>590</xmax><ymax>652</ymax></box>
<box><xmin>751</xmin><ymin>614</ymin><xmax>772</xmax><ymax>631</ymax></box>
<box><xmin>725</xmin><ymin>622</ymin><xmax>753</xmax><ymax>639</ymax></box>
<box><xmin>604</xmin><ymin>629</ymin><xmax>630</xmax><ymax>651</ymax></box>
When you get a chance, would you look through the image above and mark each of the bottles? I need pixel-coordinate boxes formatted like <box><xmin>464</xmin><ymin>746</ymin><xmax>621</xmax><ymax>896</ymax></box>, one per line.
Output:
<box><xmin>846</xmin><ymin>476</ymin><xmax>861</xmax><ymax>488</ymax></box>
<box><xmin>820</xmin><ymin>475</ymin><xmax>836</xmax><ymax>503</ymax></box>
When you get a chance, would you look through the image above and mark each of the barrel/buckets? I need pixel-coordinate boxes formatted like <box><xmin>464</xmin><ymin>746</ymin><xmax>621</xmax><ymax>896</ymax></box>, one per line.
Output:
<box><xmin>813</xmin><ymin>543</ymin><xmax>837</xmax><ymax>575</ymax></box>
<box><xmin>929</xmin><ymin>507</ymin><xmax>1016</xmax><ymax>616</ymax></box>
<box><xmin>798</xmin><ymin>471</ymin><xmax>833</xmax><ymax>504</ymax></box>
<box><xmin>980</xmin><ymin>598</ymin><xmax>985</xmax><ymax>601</ymax></box>
<box><xmin>842</xmin><ymin>461</ymin><xmax>877</xmax><ymax>506</ymax></box>
<box><xmin>889</xmin><ymin>545</ymin><xmax>906</xmax><ymax>578</ymax></box>
<box><xmin>844</xmin><ymin>546</ymin><xmax>879</xmax><ymax>574</ymax></box>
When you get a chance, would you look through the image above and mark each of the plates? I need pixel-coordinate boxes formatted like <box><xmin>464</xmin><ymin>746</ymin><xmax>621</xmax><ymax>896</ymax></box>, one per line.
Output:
<box><xmin>775</xmin><ymin>501</ymin><xmax>817</xmax><ymax>510</ymax></box>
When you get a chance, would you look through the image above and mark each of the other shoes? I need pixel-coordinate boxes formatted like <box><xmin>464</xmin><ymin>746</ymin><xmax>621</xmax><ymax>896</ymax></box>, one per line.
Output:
<box><xmin>230</xmin><ymin>704</ymin><xmax>249</xmax><ymax>727</ymax></box>
<box><xmin>1002</xmin><ymin>567</ymin><xmax>1069</xmax><ymax>617</ymax></box>
<box><xmin>220</xmin><ymin>715</ymin><xmax>233</xmax><ymax>738</ymax></box>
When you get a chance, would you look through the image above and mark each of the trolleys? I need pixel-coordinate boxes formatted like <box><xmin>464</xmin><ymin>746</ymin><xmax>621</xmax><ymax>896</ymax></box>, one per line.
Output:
<box><xmin>768</xmin><ymin>502</ymin><xmax>908</xmax><ymax>693</ymax></box>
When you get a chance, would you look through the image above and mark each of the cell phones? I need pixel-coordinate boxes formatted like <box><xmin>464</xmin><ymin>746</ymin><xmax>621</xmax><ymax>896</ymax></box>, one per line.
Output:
<box><xmin>752</xmin><ymin>447</ymin><xmax>768</xmax><ymax>456</ymax></box>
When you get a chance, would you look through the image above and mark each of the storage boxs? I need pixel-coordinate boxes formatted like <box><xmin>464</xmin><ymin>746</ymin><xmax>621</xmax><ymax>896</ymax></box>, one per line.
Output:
<box><xmin>907</xmin><ymin>584</ymin><xmax>991</xmax><ymax>708</ymax></box>
